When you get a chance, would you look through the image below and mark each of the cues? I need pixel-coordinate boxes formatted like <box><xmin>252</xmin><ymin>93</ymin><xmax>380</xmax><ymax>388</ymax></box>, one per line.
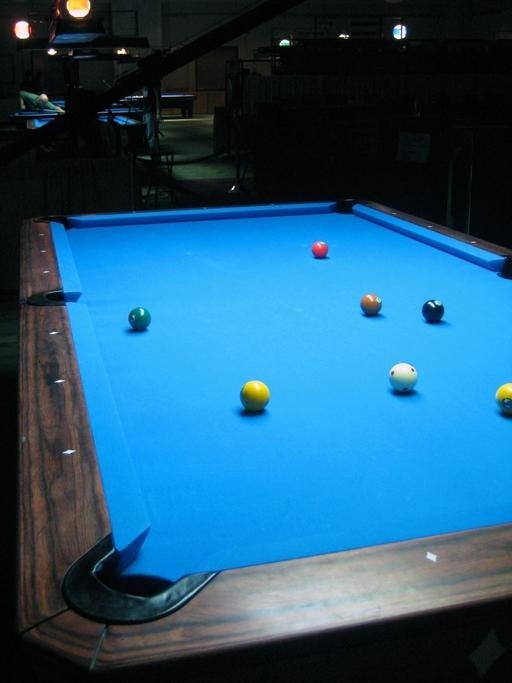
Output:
<box><xmin>25</xmin><ymin>107</ymin><xmax>63</xmax><ymax>113</ymax></box>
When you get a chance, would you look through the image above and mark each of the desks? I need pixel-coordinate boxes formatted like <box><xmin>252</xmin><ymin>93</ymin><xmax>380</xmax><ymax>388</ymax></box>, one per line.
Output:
<box><xmin>8</xmin><ymin>94</ymin><xmax>196</xmax><ymax>139</ymax></box>
<box><xmin>19</xmin><ymin>193</ymin><xmax>512</xmax><ymax>683</ymax></box>
